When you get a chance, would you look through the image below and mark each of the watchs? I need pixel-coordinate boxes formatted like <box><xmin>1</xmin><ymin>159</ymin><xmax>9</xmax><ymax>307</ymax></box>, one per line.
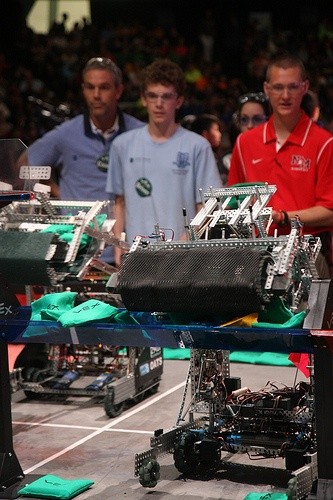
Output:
<box><xmin>278</xmin><ymin>210</ymin><xmax>288</xmax><ymax>227</ymax></box>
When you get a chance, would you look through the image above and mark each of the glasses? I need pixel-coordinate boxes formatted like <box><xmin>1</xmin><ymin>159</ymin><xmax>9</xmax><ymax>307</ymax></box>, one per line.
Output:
<box><xmin>238</xmin><ymin>93</ymin><xmax>265</xmax><ymax>104</ymax></box>
<box><xmin>240</xmin><ymin>115</ymin><xmax>268</xmax><ymax>125</ymax></box>
<box><xmin>269</xmin><ymin>82</ymin><xmax>303</xmax><ymax>92</ymax></box>
<box><xmin>88</xmin><ymin>57</ymin><xmax>116</xmax><ymax>67</ymax></box>
<box><xmin>145</xmin><ymin>92</ymin><xmax>178</xmax><ymax>100</ymax></box>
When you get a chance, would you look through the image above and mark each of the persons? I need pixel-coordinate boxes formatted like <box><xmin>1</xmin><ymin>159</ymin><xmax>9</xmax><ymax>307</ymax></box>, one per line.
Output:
<box><xmin>106</xmin><ymin>60</ymin><xmax>223</xmax><ymax>267</ymax></box>
<box><xmin>182</xmin><ymin>91</ymin><xmax>333</xmax><ymax>188</ymax></box>
<box><xmin>227</xmin><ymin>55</ymin><xmax>333</xmax><ymax>314</ymax></box>
<box><xmin>16</xmin><ymin>59</ymin><xmax>146</xmax><ymax>270</ymax></box>
